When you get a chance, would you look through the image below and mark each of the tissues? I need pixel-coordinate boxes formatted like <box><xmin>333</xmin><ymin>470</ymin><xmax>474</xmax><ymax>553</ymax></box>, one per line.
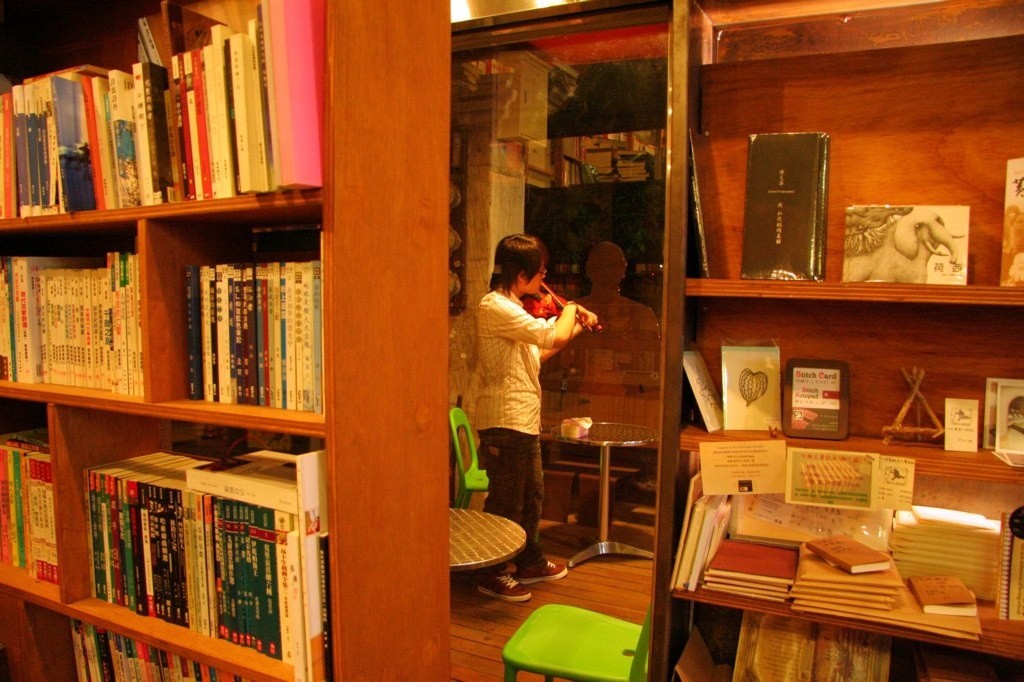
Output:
<box><xmin>560</xmin><ymin>417</ymin><xmax>593</xmax><ymax>439</ymax></box>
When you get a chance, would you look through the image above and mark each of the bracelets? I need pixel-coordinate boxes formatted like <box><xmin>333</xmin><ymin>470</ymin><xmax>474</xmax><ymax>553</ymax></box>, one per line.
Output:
<box><xmin>566</xmin><ymin>301</ymin><xmax>579</xmax><ymax>314</ymax></box>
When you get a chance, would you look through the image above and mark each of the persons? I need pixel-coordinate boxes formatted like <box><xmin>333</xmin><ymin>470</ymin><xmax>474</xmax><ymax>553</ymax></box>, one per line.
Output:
<box><xmin>474</xmin><ymin>233</ymin><xmax>599</xmax><ymax>602</ymax></box>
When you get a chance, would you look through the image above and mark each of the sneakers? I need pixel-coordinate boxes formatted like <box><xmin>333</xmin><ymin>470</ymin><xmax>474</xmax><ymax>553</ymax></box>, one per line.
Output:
<box><xmin>517</xmin><ymin>560</ymin><xmax>568</xmax><ymax>584</ymax></box>
<box><xmin>476</xmin><ymin>571</ymin><xmax>532</xmax><ymax>602</ymax></box>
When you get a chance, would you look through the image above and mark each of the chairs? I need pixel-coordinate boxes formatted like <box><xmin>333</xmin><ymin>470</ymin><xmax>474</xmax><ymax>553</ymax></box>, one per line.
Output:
<box><xmin>501</xmin><ymin>603</ymin><xmax>651</xmax><ymax>682</ymax></box>
<box><xmin>449</xmin><ymin>408</ymin><xmax>488</xmax><ymax>510</ymax></box>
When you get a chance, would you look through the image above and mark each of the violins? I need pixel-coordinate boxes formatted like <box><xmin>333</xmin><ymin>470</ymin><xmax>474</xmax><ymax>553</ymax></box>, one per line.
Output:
<box><xmin>519</xmin><ymin>291</ymin><xmax>605</xmax><ymax>335</ymax></box>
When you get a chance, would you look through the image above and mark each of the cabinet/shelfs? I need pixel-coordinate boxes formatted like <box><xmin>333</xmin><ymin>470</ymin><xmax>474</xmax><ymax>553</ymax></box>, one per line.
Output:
<box><xmin>0</xmin><ymin>0</ymin><xmax>453</xmax><ymax>682</ymax></box>
<box><xmin>647</xmin><ymin>0</ymin><xmax>1024</xmax><ymax>682</ymax></box>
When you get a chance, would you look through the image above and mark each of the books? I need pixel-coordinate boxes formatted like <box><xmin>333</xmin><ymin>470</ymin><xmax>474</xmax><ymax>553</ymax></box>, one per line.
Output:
<box><xmin>669</xmin><ymin>471</ymin><xmax>983</xmax><ymax>643</ymax></box>
<box><xmin>841</xmin><ymin>204</ymin><xmax>969</xmax><ymax>287</ymax></box>
<box><xmin>731</xmin><ymin>610</ymin><xmax>892</xmax><ymax>682</ymax></box>
<box><xmin>0</xmin><ymin>251</ymin><xmax>322</xmax><ymax>416</ymax></box>
<box><xmin>994</xmin><ymin>513</ymin><xmax>1024</xmax><ymax>622</ymax></box>
<box><xmin>740</xmin><ymin>132</ymin><xmax>829</xmax><ymax>283</ymax></box>
<box><xmin>69</xmin><ymin>449</ymin><xmax>334</xmax><ymax>682</ymax></box>
<box><xmin>1000</xmin><ymin>158</ymin><xmax>1024</xmax><ymax>286</ymax></box>
<box><xmin>0</xmin><ymin>0</ymin><xmax>326</xmax><ymax>219</ymax></box>
<box><xmin>553</xmin><ymin>137</ymin><xmax>654</xmax><ymax>188</ymax></box>
<box><xmin>0</xmin><ymin>426</ymin><xmax>59</xmax><ymax>587</ymax></box>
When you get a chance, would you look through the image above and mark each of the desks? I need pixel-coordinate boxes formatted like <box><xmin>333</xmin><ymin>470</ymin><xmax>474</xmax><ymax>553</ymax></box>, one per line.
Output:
<box><xmin>551</xmin><ymin>422</ymin><xmax>659</xmax><ymax>569</ymax></box>
<box><xmin>448</xmin><ymin>508</ymin><xmax>528</xmax><ymax>569</ymax></box>
